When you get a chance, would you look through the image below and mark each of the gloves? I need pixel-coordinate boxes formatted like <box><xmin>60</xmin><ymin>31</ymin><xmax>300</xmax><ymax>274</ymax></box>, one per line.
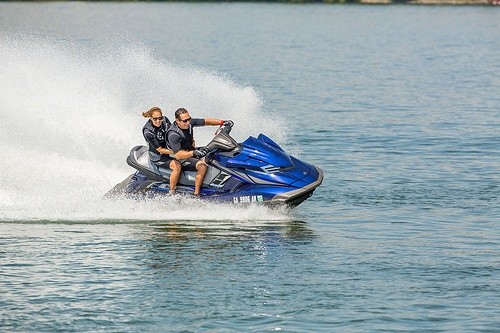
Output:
<box><xmin>223</xmin><ymin>120</ymin><xmax>234</xmax><ymax>127</ymax></box>
<box><xmin>193</xmin><ymin>149</ymin><xmax>205</xmax><ymax>158</ymax></box>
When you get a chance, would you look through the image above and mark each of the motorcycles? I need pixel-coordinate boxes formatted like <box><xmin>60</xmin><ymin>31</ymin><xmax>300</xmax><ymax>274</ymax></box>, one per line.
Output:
<box><xmin>102</xmin><ymin>118</ymin><xmax>325</xmax><ymax>214</ymax></box>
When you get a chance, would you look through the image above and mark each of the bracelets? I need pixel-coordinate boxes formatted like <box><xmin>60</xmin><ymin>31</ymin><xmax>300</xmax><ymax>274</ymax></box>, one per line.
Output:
<box><xmin>220</xmin><ymin>120</ymin><xmax>224</xmax><ymax>124</ymax></box>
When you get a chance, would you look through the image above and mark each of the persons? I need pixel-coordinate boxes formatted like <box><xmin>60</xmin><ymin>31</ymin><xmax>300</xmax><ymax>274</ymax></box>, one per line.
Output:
<box><xmin>165</xmin><ymin>108</ymin><xmax>235</xmax><ymax>197</ymax></box>
<box><xmin>142</xmin><ymin>106</ymin><xmax>182</xmax><ymax>196</ymax></box>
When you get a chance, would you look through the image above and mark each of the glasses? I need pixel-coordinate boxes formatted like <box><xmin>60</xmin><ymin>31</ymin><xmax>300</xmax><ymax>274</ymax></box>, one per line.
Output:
<box><xmin>151</xmin><ymin>116</ymin><xmax>163</xmax><ymax>121</ymax></box>
<box><xmin>179</xmin><ymin>116</ymin><xmax>191</xmax><ymax>123</ymax></box>
